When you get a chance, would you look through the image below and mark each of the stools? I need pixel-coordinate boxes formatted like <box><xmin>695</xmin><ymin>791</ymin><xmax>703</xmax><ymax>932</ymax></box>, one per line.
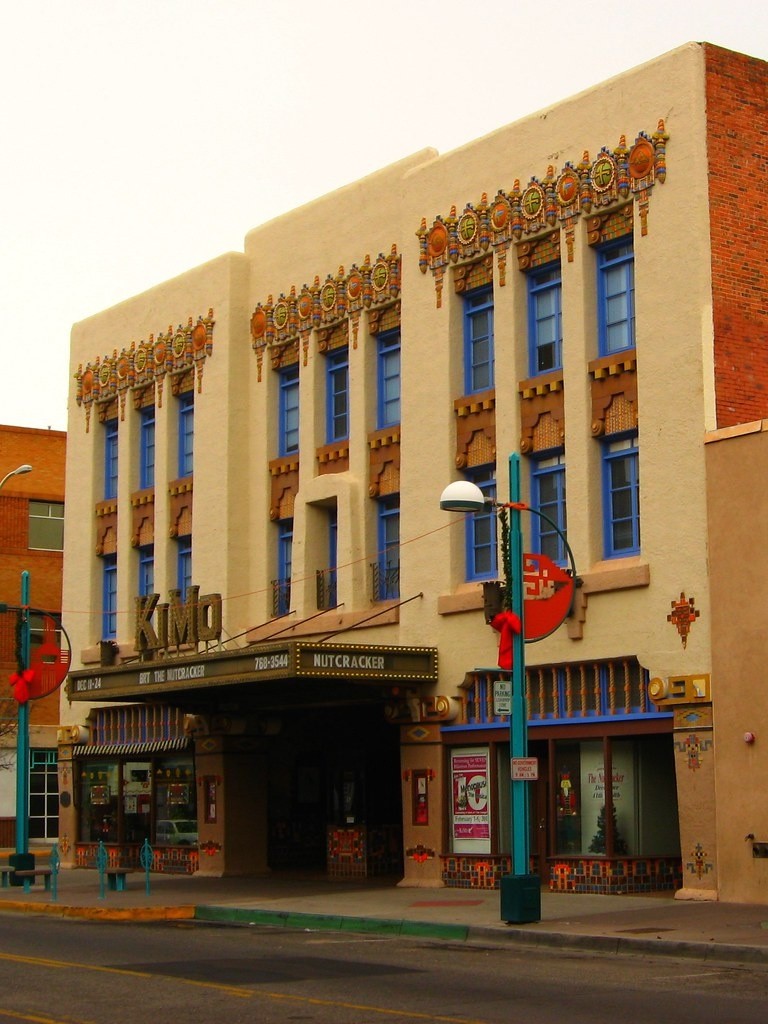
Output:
<box><xmin>0</xmin><ymin>865</ymin><xmax>15</xmax><ymax>889</ymax></box>
<box><xmin>15</xmin><ymin>868</ymin><xmax>59</xmax><ymax>895</ymax></box>
<box><xmin>104</xmin><ymin>867</ymin><xmax>135</xmax><ymax>891</ymax></box>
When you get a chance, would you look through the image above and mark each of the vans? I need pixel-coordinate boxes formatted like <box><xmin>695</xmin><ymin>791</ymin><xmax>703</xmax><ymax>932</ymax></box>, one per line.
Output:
<box><xmin>155</xmin><ymin>819</ymin><xmax>198</xmax><ymax>846</ymax></box>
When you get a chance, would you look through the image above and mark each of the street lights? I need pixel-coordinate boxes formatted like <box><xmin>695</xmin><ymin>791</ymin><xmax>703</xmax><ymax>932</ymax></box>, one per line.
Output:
<box><xmin>440</xmin><ymin>450</ymin><xmax>577</xmax><ymax>924</ymax></box>
